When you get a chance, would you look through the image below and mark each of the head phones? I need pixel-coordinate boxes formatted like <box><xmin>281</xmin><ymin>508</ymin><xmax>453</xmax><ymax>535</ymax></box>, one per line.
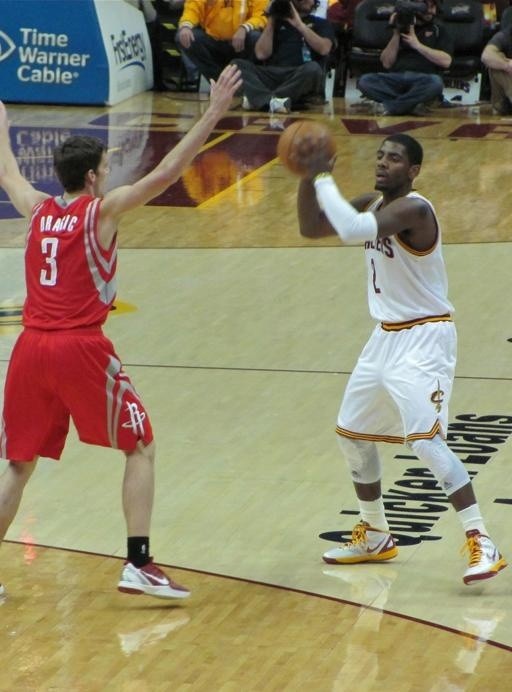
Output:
<box><xmin>433</xmin><ymin>0</ymin><xmax>445</xmax><ymax>14</ymax></box>
<box><xmin>313</xmin><ymin>0</ymin><xmax>320</xmax><ymax>10</ymax></box>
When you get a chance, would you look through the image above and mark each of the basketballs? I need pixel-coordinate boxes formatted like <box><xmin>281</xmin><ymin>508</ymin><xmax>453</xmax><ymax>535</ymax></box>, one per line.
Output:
<box><xmin>277</xmin><ymin>121</ymin><xmax>336</xmax><ymax>175</ymax></box>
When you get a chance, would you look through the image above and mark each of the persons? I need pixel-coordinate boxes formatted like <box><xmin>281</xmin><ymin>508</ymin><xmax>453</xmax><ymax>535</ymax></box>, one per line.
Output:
<box><xmin>0</xmin><ymin>63</ymin><xmax>243</xmax><ymax>600</ymax></box>
<box><xmin>120</xmin><ymin>0</ymin><xmax>512</xmax><ymax>115</ymax></box>
<box><xmin>289</xmin><ymin>134</ymin><xmax>508</xmax><ymax>584</ymax></box>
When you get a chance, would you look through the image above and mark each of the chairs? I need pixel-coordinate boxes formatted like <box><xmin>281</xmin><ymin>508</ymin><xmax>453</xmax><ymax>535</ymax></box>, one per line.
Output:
<box><xmin>434</xmin><ymin>0</ymin><xmax>487</xmax><ymax>76</ymax></box>
<box><xmin>346</xmin><ymin>0</ymin><xmax>418</xmax><ymax>80</ymax></box>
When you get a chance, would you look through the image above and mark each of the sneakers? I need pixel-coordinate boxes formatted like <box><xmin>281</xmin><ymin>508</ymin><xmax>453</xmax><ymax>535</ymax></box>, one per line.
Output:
<box><xmin>269</xmin><ymin>97</ymin><xmax>292</xmax><ymax>114</ymax></box>
<box><xmin>118</xmin><ymin>560</ymin><xmax>191</xmax><ymax>599</ymax></box>
<box><xmin>461</xmin><ymin>529</ymin><xmax>507</xmax><ymax>584</ymax></box>
<box><xmin>322</xmin><ymin>521</ymin><xmax>397</xmax><ymax>562</ymax></box>
